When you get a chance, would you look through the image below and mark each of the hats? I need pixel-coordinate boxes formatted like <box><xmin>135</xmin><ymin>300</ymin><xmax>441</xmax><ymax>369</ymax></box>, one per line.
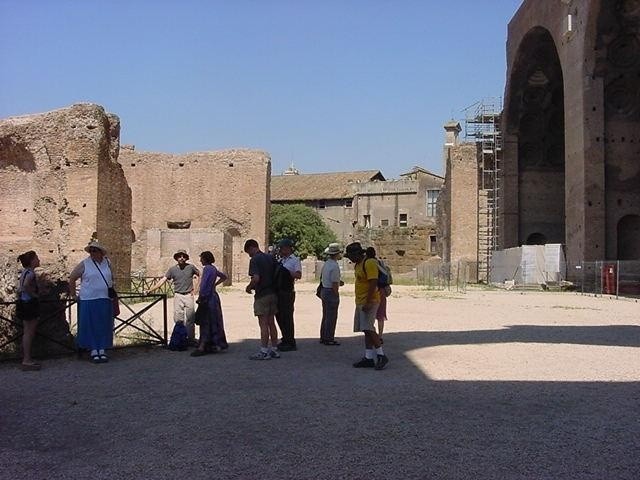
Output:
<box><xmin>173</xmin><ymin>249</ymin><xmax>189</xmax><ymax>261</ymax></box>
<box><xmin>83</xmin><ymin>241</ymin><xmax>107</xmax><ymax>255</ymax></box>
<box><xmin>276</xmin><ymin>239</ymin><xmax>293</xmax><ymax>248</ymax></box>
<box><xmin>324</xmin><ymin>243</ymin><xmax>342</xmax><ymax>256</ymax></box>
<box><xmin>343</xmin><ymin>242</ymin><xmax>367</xmax><ymax>258</ymax></box>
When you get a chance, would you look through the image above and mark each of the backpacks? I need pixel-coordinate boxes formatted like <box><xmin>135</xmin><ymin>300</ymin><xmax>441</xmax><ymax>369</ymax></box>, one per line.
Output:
<box><xmin>378</xmin><ymin>259</ymin><xmax>393</xmax><ymax>284</ymax></box>
<box><xmin>362</xmin><ymin>257</ymin><xmax>387</xmax><ymax>288</ymax></box>
<box><xmin>168</xmin><ymin>320</ymin><xmax>189</xmax><ymax>352</ymax></box>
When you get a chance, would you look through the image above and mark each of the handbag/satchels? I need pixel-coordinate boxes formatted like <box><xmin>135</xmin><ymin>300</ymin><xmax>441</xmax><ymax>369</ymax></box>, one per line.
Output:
<box><xmin>14</xmin><ymin>271</ymin><xmax>39</xmax><ymax>322</ymax></box>
<box><xmin>193</xmin><ymin>302</ymin><xmax>208</xmax><ymax>326</ymax></box>
<box><xmin>316</xmin><ymin>275</ymin><xmax>322</xmax><ymax>298</ymax></box>
<box><xmin>107</xmin><ymin>286</ymin><xmax>117</xmax><ymax>298</ymax></box>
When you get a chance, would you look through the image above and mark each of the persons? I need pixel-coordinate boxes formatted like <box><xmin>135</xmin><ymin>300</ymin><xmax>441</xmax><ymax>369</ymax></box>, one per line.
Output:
<box><xmin>366</xmin><ymin>247</ymin><xmax>388</xmax><ymax>344</ymax></box>
<box><xmin>343</xmin><ymin>242</ymin><xmax>389</xmax><ymax>369</ymax></box>
<box><xmin>70</xmin><ymin>242</ymin><xmax>115</xmax><ymax>363</ymax></box>
<box><xmin>143</xmin><ymin>249</ymin><xmax>201</xmax><ymax>348</ymax></box>
<box><xmin>17</xmin><ymin>251</ymin><xmax>41</xmax><ymax>371</ymax></box>
<box><xmin>276</xmin><ymin>239</ymin><xmax>301</xmax><ymax>351</ymax></box>
<box><xmin>320</xmin><ymin>243</ymin><xmax>343</xmax><ymax>345</ymax></box>
<box><xmin>191</xmin><ymin>251</ymin><xmax>229</xmax><ymax>356</ymax></box>
<box><xmin>244</xmin><ymin>240</ymin><xmax>280</xmax><ymax>360</ymax></box>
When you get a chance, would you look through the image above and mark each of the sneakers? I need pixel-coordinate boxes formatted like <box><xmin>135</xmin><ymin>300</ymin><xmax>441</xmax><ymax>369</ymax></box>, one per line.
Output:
<box><xmin>375</xmin><ymin>354</ymin><xmax>389</xmax><ymax>370</ymax></box>
<box><xmin>352</xmin><ymin>356</ymin><xmax>375</xmax><ymax>368</ymax></box>
<box><xmin>248</xmin><ymin>342</ymin><xmax>297</xmax><ymax>361</ymax></box>
<box><xmin>189</xmin><ymin>338</ymin><xmax>217</xmax><ymax>357</ymax></box>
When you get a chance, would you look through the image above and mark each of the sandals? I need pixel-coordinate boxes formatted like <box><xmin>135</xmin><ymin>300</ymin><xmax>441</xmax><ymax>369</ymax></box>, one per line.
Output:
<box><xmin>22</xmin><ymin>362</ymin><xmax>41</xmax><ymax>370</ymax></box>
<box><xmin>100</xmin><ymin>354</ymin><xmax>109</xmax><ymax>362</ymax></box>
<box><xmin>324</xmin><ymin>340</ymin><xmax>340</xmax><ymax>345</ymax></box>
<box><xmin>88</xmin><ymin>353</ymin><xmax>99</xmax><ymax>363</ymax></box>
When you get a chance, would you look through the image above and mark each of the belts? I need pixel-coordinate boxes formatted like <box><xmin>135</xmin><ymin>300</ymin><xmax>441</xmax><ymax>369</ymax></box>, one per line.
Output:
<box><xmin>175</xmin><ymin>289</ymin><xmax>193</xmax><ymax>295</ymax></box>
